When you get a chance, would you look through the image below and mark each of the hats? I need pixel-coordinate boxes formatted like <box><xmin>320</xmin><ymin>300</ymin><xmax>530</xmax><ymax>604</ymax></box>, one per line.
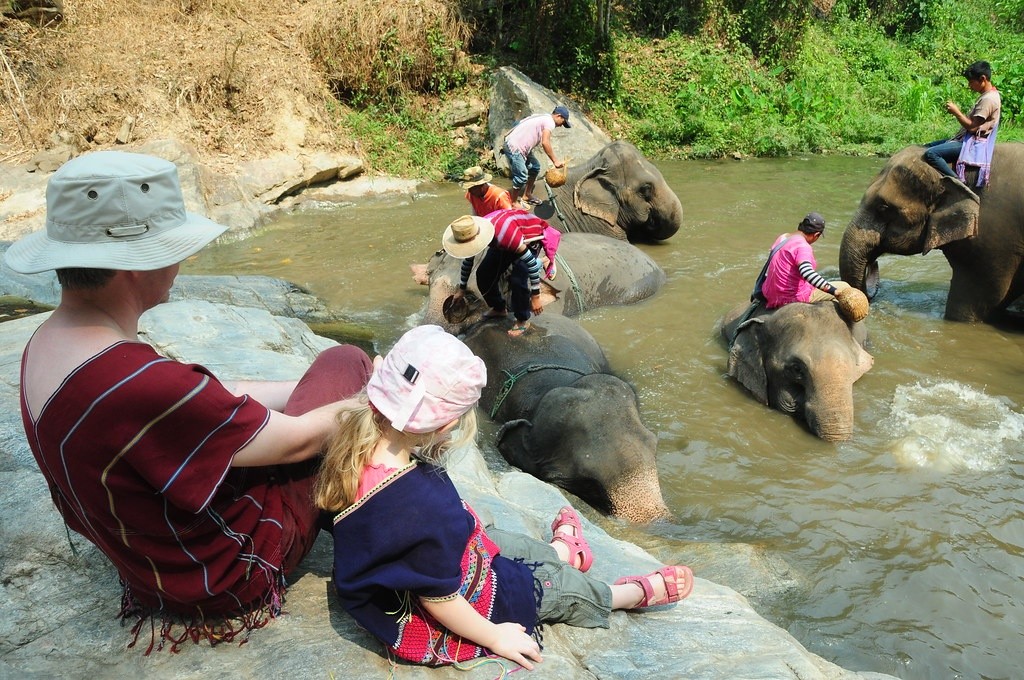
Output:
<box><xmin>5</xmin><ymin>153</ymin><xmax>232</xmax><ymax>274</ymax></box>
<box><xmin>458</xmin><ymin>166</ymin><xmax>492</xmax><ymax>189</ymax></box>
<box><xmin>554</xmin><ymin>105</ymin><xmax>571</xmax><ymax>129</ymax></box>
<box><xmin>367</xmin><ymin>324</ymin><xmax>488</xmax><ymax>434</ymax></box>
<box><xmin>442</xmin><ymin>215</ymin><xmax>495</xmax><ymax>259</ymax></box>
<box><xmin>805</xmin><ymin>212</ymin><xmax>825</xmax><ymax>239</ymax></box>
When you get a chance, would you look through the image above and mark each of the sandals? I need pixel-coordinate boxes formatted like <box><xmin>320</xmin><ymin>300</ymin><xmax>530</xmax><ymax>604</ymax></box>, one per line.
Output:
<box><xmin>551</xmin><ymin>505</ymin><xmax>593</xmax><ymax>572</ymax></box>
<box><xmin>612</xmin><ymin>566</ymin><xmax>694</xmax><ymax>609</ymax></box>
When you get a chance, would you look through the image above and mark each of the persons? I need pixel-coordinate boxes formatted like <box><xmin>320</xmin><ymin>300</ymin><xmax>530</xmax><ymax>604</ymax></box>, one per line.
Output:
<box><xmin>307</xmin><ymin>324</ymin><xmax>694</xmax><ymax>680</ymax></box>
<box><xmin>920</xmin><ymin>61</ymin><xmax>1002</xmax><ymax>186</ymax></box>
<box><xmin>442</xmin><ymin>208</ymin><xmax>561</xmax><ymax>338</ymax></box>
<box><xmin>761</xmin><ymin>211</ymin><xmax>843</xmax><ymax>307</ymax></box>
<box><xmin>457</xmin><ymin>165</ymin><xmax>513</xmax><ymax>217</ymax></box>
<box><xmin>6</xmin><ymin>150</ymin><xmax>374</xmax><ymax>655</ymax></box>
<box><xmin>499</xmin><ymin>106</ymin><xmax>571</xmax><ymax>211</ymax></box>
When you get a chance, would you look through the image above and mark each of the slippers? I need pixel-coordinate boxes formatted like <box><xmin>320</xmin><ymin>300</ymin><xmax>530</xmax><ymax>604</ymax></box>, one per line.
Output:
<box><xmin>521</xmin><ymin>197</ymin><xmax>542</xmax><ymax>205</ymax></box>
<box><xmin>515</xmin><ymin>200</ymin><xmax>530</xmax><ymax>210</ymax></box>
<box><xmin>507</xmin><ymin>322</ymin><xmax>530</xmax><ymax>338</ymax></box>
<box><xmin>482</xmin><ymin>310</ymin><xmax>508</xmax><ymax>318</ymax></box>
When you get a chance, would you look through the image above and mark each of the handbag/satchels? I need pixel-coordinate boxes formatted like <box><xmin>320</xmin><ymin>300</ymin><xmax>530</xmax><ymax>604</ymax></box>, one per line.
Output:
<box><xmin>750</xmin><ymin>276</ymin><xmax>767</xmax><ymax>304</ymax></box>
<box><xmin>956</xmin><ymin>135</ymin><xmax>993</xmax><ymax>188</ymax></box>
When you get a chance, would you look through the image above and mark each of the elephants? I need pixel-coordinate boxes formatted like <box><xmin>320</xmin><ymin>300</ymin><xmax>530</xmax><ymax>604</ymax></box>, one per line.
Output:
<box><xmin>837</xmin><ymin>144</ymin><xmax>1024</xmax><ymax>322</ymax></box>
<box><xmin>414</xmin><ymin>233</ymin><xmax>668</xmax><ymax>337</ymax></box>
<box><xmin>721</xmin><ymin>279</ymin><xmax>872</xmax><ymax>443</ymax></box>
<box><xmin>502</xmin><ymin>139</ymin><xmax>684</xmax><ymax>243</ymax></box>
<box><xmin>465</xmin><ymin>309</ymin><xmax>673</xmax><ymax>527</ymax></box>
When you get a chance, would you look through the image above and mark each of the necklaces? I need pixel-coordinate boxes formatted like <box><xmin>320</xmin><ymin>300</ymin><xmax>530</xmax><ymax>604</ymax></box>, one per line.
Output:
<box><xmin>950</xmin><ymin>86</ymin><xmax>994</xmax><ymax>141</ymax></box>
